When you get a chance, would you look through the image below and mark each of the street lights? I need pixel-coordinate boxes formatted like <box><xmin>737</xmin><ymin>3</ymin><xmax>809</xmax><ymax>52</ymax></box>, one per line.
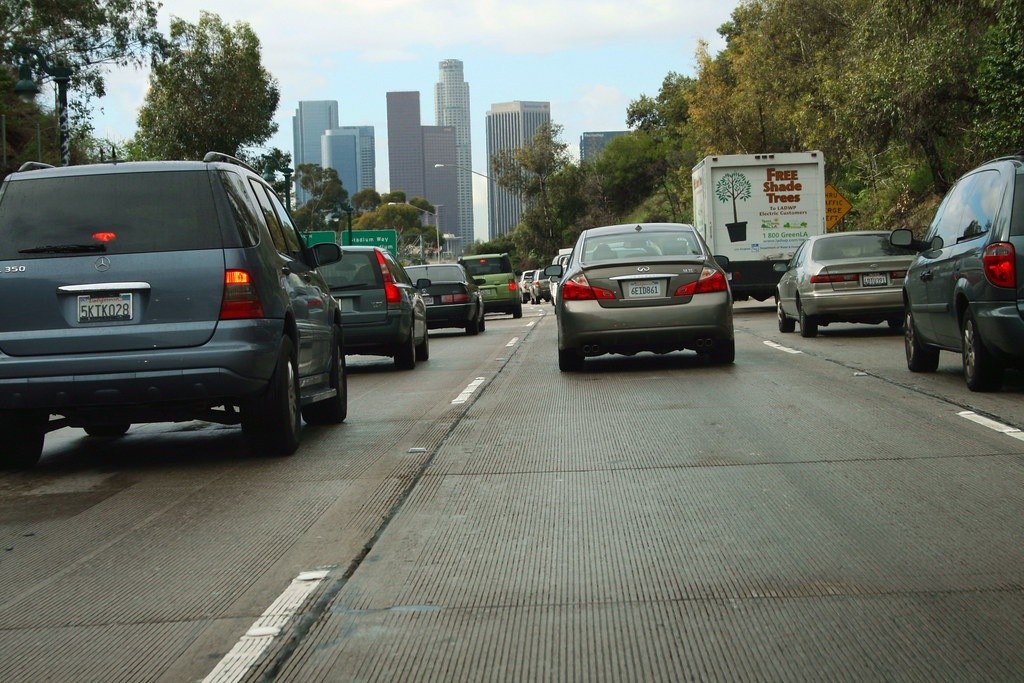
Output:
<box><xmin>388</xmin><ymin>202</ymin><xmax>440</xmax><ymax>265</ymax></box>
<box><xmin>9</xmin><ymin>44</ymin><xmax>74</xmax><ymax>167</ymax></box>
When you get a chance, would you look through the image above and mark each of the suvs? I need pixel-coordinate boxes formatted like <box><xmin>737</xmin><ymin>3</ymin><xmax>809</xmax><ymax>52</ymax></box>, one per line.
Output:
<box><xmin>890</xmin><ymin>154</ymin><xmax>1024</xmax><ymax>391</ymax></box>
<box><xmin>1</xmin><ymin>151</ymin><xmax>346</xmax><ymax>472</ymax></box>
<box><xmin>518</xmin><ymin>248</ymin><xmax>574</xmax><ymax>306</ymax></box>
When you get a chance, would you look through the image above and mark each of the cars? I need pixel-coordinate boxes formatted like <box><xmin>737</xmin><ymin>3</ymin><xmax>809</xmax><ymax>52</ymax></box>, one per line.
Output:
<box><xmin>456</xmin><ymin>253</ymin><xmax>523</xmax><ymax>319</ymax></box>
<box><xmin>319</xmin><ymin>245</ymin><xmax>430</xmax><ymax>369</ymax></box>
<box><xmin>555</xmin><ymin>223</ymin><xmax>736</xmax><ymax>372</ymax></box>
<box><xmin>772</xmin><ymin>230</ymin><xmax>919</xmax><ymax>338</ymax></box>
<box><xmin>402</xmin><ymin>262</ymin><xmax>486</xmax><ymax>336</ymax></box>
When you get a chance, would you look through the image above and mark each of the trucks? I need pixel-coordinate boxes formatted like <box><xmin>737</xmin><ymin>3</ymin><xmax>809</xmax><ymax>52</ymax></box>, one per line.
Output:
<box><xmin>692</xmin><ymin>150</ymin><xmax>826</xmax><ymax>305</ymax></box>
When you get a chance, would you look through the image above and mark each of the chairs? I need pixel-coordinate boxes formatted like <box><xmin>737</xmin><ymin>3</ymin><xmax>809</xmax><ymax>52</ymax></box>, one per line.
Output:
<box><xmin>818</xmin><ymin>247</ymin><xmax>843</xmax><ymax>259</ymax></box>
<box><xmin>591</xmin><ymin>250</ymin><xmax>618</xmax><ymax>260</ymax></box>
<box><xmin>353</xmin><ymin>264</ymin><xmax>374</xmax><ymax>281</ymax></box>
